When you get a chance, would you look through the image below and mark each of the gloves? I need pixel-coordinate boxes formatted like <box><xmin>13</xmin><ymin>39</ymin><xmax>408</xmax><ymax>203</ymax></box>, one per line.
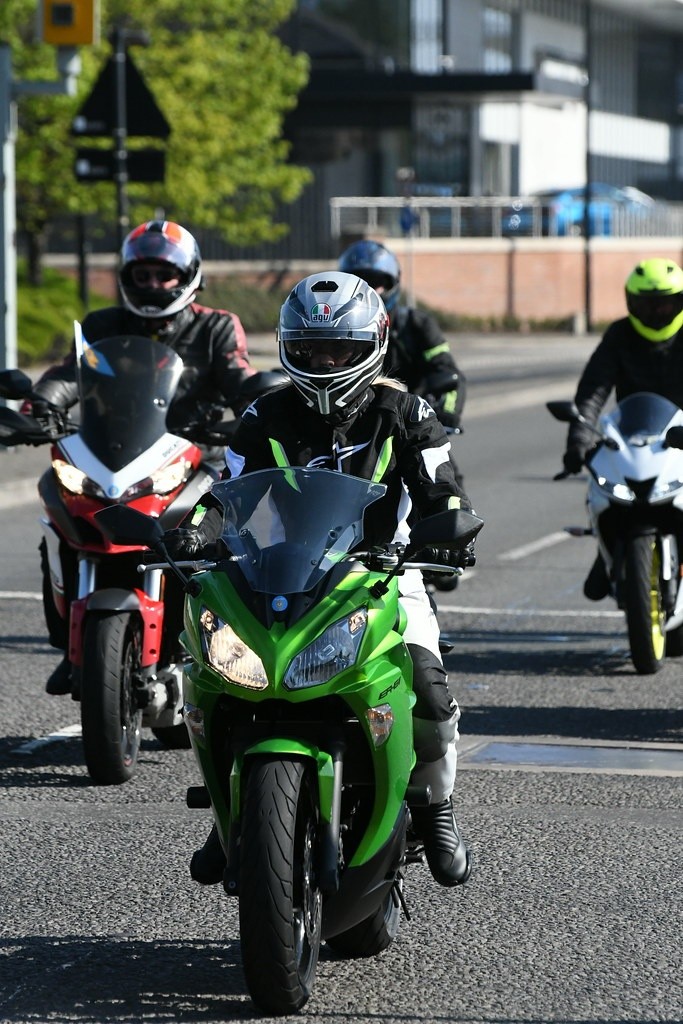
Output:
<box><xmin>420</xmin><ymin>548</ymin><xmax>476</xmax><ymax>577</ymax></box>
<box><xmin>158</xmin><ymin>527</ymin><xmax>208</xmax><ymax>565</ymax></box>
<box><xmin>561</xmin><ymin>440</ymin><xmax>590</xmax><ymax>470</ymax></box>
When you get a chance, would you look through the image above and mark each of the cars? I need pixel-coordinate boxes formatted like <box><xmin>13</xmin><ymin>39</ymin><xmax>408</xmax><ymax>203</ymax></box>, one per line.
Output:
<box><xmin>498</xmin><ymin>183</ymin><xmax>663</xmax><ymax>239</ymax></box>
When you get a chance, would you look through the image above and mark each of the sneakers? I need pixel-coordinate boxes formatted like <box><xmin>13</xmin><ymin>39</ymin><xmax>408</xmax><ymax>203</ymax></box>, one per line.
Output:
<box><xmin>416</xmin><ymin>799</ymin><xmax>468</xmax><ymax>887</ymax></box>
<box><xmin>46</xmin><ymin>658</ymin><xmax>75</xmax><ymax>697</ymax></box>
<box><xmin>187</xmin><ymin>820</ymin><xmax>228</xmax><ymax>887</ymax></box>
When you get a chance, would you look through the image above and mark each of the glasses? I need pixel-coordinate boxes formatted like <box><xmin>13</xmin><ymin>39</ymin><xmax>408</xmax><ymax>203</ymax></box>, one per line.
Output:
<box><xmin>367</xmin><ymin>276</ymin><xmax>384</xmax><ymax>289</ymax></box>
<box><xmin>284</xmin><ymin>340</ymin><xmax>362</xmax><ymax>358</ymax></box>
<box><xmin>133</xmin><ymin>268</ymin><xmax>180</xmax><ymax>283</ymax></box>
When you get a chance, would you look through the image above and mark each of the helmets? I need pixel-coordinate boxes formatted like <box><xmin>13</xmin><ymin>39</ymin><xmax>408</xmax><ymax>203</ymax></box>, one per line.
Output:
<box><xmin>116</xmin><ymin>220</ymin><xmax>206</xmax><ymax>316</ymax></box>
<box><xmin>624</xmin><ymin>257</ymin><xmax>683</xmax><ymax>342</ymax></box>
<box><xmin>276</xmin><ymin>271</ymin><xmax>390</xmax><ymax>416</ymax></box>
<box><xmin>338</xmin><ymin>239</ymin><xmax>400</xmax><ymax>311</ymax></box>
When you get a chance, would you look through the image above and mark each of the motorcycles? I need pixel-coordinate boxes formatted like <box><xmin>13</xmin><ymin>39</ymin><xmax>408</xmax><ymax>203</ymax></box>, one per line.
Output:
<box><xmin>91</xmin><ymin>464</ymin><xmax>488</xmax><ymax>1019</ymax></box>
<box><xmin>544</xmin><ymin>389</ymin><xmax>683</xmax><ymax>678</ymax></box>
<box><xmin>0</xmin><ymin>334</ymin><xmax>289</xmax><ymax>789</ymax></box>
<box><xmin>268</xmin><ymin>364</ymin><xmax>478</xmax><ymax>616</ymax></box>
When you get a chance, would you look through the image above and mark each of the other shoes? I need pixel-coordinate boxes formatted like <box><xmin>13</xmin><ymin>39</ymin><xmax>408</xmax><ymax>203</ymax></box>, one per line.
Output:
<box><xmin>582</xmin><ymin>550</ymin><xmax>610</xmax><ymax>601</ymax></box>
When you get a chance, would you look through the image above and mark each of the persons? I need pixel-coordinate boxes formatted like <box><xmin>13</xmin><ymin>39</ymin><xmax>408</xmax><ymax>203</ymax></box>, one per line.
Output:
<box><xmin>171</xmin><ymin>270</ymin><xmax>476</xmax><ymax>890</ymax></box>
<box><xmin>337</xmin><ymin>237</ymin><xmax>469</xmax><ymax>440</ymax></box>
<box><xmin>17</xmin><ymin>219</ymin><xmax>263</xmax><ymax>702</ymax></box>
<box><xmin>563</xmin><ymin>257</ymin><xmax>683</xmax><ymax>601</ymax></box>
